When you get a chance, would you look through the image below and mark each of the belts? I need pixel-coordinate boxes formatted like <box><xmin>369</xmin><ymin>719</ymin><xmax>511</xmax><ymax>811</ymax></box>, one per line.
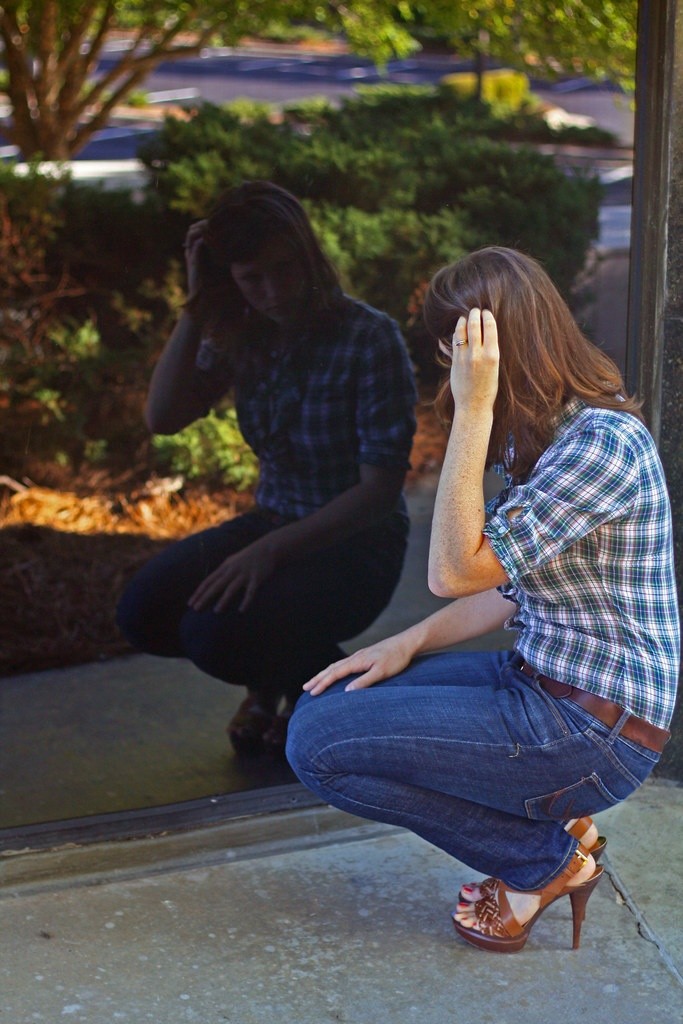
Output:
<box><xmin>518</xmin><ymin>658</ymin><xmax>670</xmax><ymax>753</ymax></box>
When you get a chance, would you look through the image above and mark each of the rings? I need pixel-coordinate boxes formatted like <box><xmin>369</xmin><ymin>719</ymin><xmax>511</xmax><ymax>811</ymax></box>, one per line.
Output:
<box><xmin>454</xmin><ymin>340</ymin><xmax>466</xmax><ymax>346</ymax></box>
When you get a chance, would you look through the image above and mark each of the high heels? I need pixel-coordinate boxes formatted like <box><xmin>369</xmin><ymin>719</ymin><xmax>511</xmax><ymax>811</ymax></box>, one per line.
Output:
<box><xmin>228</xmin><ymin>702</ymin><xmax>289</xmax><ymax>756</ymax></box>
<box><xmin>449</xmin><ymin>812</ymin><xmax>607</xmax><ymax>952</ymax></box>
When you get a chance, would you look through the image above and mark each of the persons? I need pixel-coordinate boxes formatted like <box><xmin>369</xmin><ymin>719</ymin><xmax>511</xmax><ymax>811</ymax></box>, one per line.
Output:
<box><xmin>136</xmin><ymin>182</ymin><xmax>419</xmax><ymax>798</ymax></box>
<box><xmin>284</xmin><ymin>241</ymin><xmax>682</xmax><ymax>950</ymax></box>
